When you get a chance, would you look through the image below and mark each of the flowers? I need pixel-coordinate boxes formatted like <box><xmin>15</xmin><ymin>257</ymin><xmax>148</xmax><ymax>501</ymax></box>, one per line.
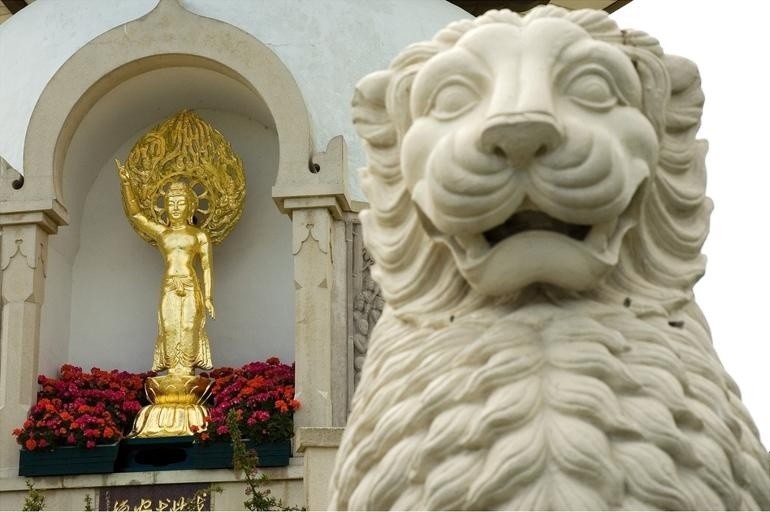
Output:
<box><xmin>12</xmin><ymin>356</ymin><xmax>301</xmax><ymax>449</ymax></box>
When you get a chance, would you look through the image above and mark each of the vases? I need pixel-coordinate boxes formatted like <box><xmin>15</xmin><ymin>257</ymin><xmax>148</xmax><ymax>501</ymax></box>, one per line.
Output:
<box><xmin>18</xmin><ymin>441</ymin><xmax>291</xmax><ymax>475</ymax></box>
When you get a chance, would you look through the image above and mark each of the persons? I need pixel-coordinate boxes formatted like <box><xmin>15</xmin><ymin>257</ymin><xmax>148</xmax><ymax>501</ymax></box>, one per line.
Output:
<box><xmin>113</xmin><ymin>148</ymin><xmax>247</xmax><ymax>378</ymax></box>
